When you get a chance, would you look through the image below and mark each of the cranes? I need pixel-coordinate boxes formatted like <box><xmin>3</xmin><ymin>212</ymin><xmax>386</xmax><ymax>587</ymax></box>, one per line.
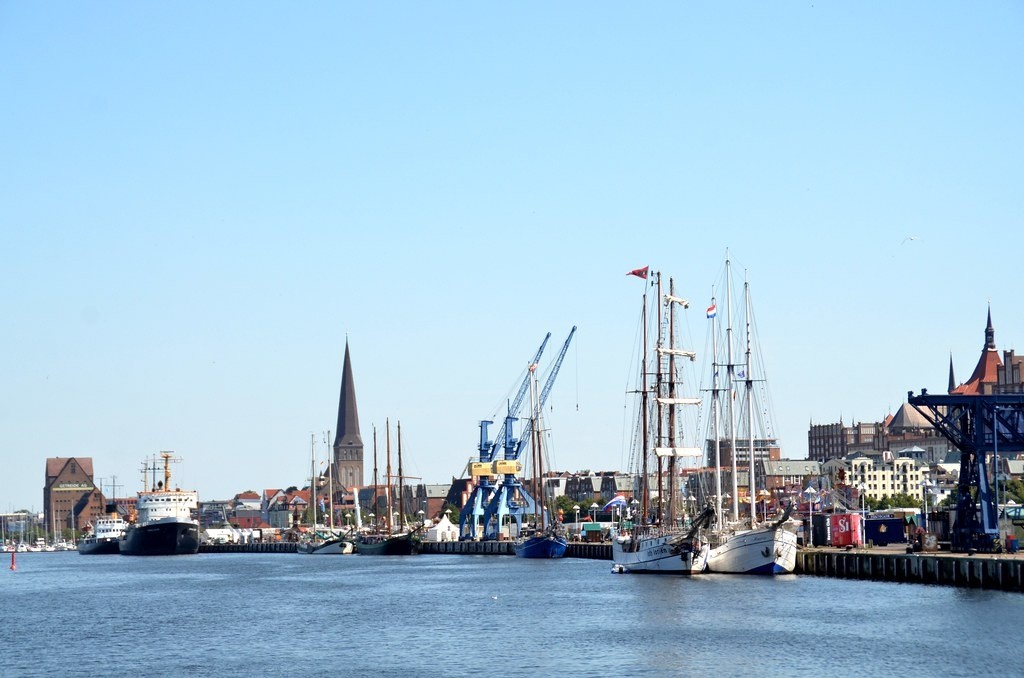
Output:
<box><xmin>459</xmin><ymin>325</ymin><xmax>579</xmax><ymax>541</ymax></box>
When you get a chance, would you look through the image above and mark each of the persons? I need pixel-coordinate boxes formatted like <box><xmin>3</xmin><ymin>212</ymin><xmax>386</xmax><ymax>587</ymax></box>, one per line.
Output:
<box><xmin>553</xmin><ymin>533</ymin><xmax>559</xmax><ymax>540</ymax></box>
<box><xmin>547</xmin><ymin>534</ymin><xmax>551</xmax><ymax>539</ymax></box>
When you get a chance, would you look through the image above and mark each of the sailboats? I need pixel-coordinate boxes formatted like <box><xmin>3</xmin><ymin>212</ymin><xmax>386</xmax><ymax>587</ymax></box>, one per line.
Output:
<box><xmin>350</xmin><ymin>417</ymin><xmax>425</xmax><ymax>555</ymax></box>
<box><xmin>706</xmin><ymin>247</ymin><xmax>797</xmax><ymax>576</ymax></box>
<box><xmin>513</xmin><ymin>361</ymin><xmax>567</xmax><ymax>559</ymax></box>
<box><xmin>0</xmin><ymin>509</ymin><xmax>79</xmax><ymax>552</ymax></box>
<box><xmin>609</xmin><ymin>271</ymin><xmax>711</xmax><ymax>575</ymax></box>
<box><xmin>296</xmin><ymin>430</ymin><xmax>353</xmax><ymax>554</ymax></box>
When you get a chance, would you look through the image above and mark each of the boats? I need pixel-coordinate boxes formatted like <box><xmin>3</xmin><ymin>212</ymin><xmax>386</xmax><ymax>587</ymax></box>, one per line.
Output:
<box><xmin>117</xmin><ymin>453</ymin><xmax>200</xmax><ymax>555</ymax></box>
<box><xmin>77</xmin><ymin>477</ymin><xmax>130</xmax><ymax>554</ymax></box>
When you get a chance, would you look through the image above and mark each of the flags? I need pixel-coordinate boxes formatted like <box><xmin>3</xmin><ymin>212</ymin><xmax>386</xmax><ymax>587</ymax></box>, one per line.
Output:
<box><xmin>715</xmin><ymin>372</ymin><xmax>718</xmax><ymax>376</ymax></box>
<box><xmin>738</xmin><ymin>371</ymin><xmax>745</xmax><ymax>378</ymax></box>
<box><xmin>626</xmin><ymin>265</ymin><xmax>648</xmax><ymax>278</ymax></box>
<box><xmin>707</xmin><ymin>305</ymin><xmax>717</xmax><ymax>319</ymax></box>
<box><xmin>602</xmin><ymin>495</ymin><xmax>626</xmax><ymax>513</ymax></box>
